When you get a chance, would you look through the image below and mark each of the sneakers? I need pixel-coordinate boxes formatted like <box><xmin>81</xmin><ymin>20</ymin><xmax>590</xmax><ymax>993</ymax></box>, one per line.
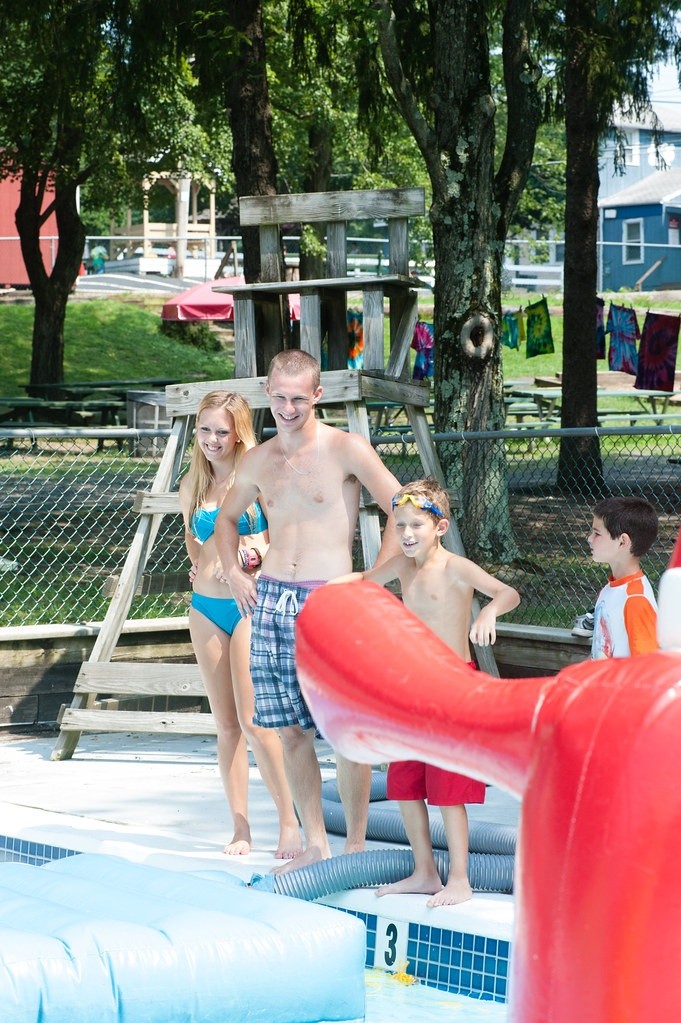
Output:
<box><xmin>571</xmin><ymin>607</ymin><xmax>596</xmax><ymax>637</ymax></box>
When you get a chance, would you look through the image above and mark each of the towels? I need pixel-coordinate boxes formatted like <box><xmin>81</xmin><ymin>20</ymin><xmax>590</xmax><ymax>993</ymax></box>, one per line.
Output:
<box><xmin>595</xmin><ymin>297</ymin><xmax>605</xmax><ymax>359</ymax></box>
<box><xmin>321</xmin><ymin>310</ymin><xmax>363</xmax><ymax>372</ymax></box>
<box><xmin>524</xmin><ymin>297</ymin><xmax>554</xmax><ymax>359</ymax></box>
<box><xmin>632</xmin><ymin>312</ymin><xmax>681</xmax><ymax>392</ymax></box>
<box><xmin>410</xmin><ymin>322</ymin><xmax>435</xmax><ymax>380</ymax></box>
<box><xmin>605</xmin><ymin>304</ymin><xmax>640</xmax><ymax>376</ymax></box>
<box><xmin>501</xmin><ymin>311</ymin><xmax>526</xmax><ymax>349</ymax></box>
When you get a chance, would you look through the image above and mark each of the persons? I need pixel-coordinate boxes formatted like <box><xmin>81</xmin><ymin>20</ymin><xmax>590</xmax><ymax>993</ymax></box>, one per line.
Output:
<box><xmin>91</xmin><ymin>242</ymin><xmax>109</xmax><ymax>274</ymax></box>
<box><xmin>325</xmin><ymin>474</ymin><xmax>521</xmax><ymax>908</ymax></box>
<box><xmin>214</xmin><ymin>349</ymin><xmax>402</xmax><ymax>878</ymax></box>
<box><xmin>179</xmin><ymin>391</ymin><xmax>300</xmax><ymax>860</ymax></box>
<box><xmin>587</xmin><ymin>496</ymin><xmax>659</xmax><ymax>659</ymax></box>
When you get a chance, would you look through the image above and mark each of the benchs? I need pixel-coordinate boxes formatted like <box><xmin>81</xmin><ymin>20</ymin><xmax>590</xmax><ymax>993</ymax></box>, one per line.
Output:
<box><xmin>213</xmin><ymin>186</ymin><xmax>424</xmax><ymax>378</ymax></box>
<box><xmin>363</xmin><ymin>398</ymin><xmax>681</xmax><ymax>439</ymax></box>
<box><xmin>0</xmin><ymin>388</ymin><xmax>149</xmax><ymax>454</ymax></box>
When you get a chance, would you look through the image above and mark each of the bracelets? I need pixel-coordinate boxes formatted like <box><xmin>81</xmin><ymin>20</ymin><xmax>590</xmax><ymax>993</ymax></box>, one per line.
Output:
<box><xmin>251</xmin><ymin>548</ymin><xmax>262</xmax><ymax>568</ymax></box>
<box><xmin>239</xmin><ymin>550</ymin><xmax>248</xmax><ymax>569</ymax></box>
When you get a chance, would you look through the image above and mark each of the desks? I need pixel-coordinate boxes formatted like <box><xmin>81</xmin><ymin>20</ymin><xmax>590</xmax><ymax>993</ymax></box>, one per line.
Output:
<box><xmin>16</xmin><ymin>378</ymin><xmax>180</xmax><ymax>419</ymax></box>
<box><xmin>0</xmin><ymin>401</ymin><xmax>127</xmax><ymax>453</ymax></box>
<box><xmin>365</xmin><ymin>379</ymin><xmax>672</xmax><ymax>438</ymax></box>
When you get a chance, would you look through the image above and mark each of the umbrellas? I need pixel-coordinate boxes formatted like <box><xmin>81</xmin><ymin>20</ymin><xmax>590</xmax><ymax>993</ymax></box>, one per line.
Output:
<box><xmin>162</xmin><ymin>275</ymin><xmax>301</xmax><ymax>321</ymax></box>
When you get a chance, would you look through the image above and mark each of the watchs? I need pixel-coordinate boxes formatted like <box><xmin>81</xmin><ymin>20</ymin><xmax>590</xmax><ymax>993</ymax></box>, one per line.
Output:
<box><xmin>248</xmin><ymin>549</ymin><xmax>258</xmax><ymax>569</ymax></box>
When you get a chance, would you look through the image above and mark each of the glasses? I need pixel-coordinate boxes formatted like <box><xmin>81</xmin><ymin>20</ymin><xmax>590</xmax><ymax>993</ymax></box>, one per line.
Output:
<box><xmin>392</xmin><ymin>493</ymin><xmax>444</xmax><ymax>519</ymax></box>
